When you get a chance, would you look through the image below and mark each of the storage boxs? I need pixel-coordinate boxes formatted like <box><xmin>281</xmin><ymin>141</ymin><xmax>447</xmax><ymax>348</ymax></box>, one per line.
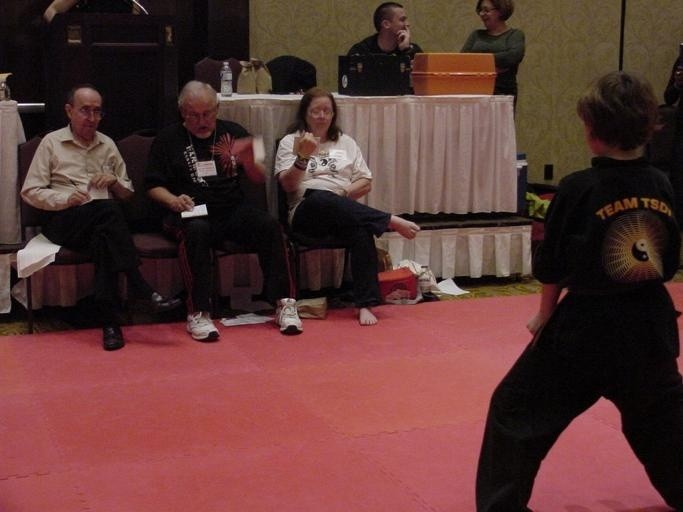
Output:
<box><xmin>412</xmin><ymin>50</ymin><xmax>497</xmax><ymax>98</ymax></box>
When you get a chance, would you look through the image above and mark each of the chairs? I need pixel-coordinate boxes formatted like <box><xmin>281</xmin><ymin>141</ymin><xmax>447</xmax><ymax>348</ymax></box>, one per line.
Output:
<box><xmin>15</xmin><ymin>133</ymin><xmax>104</xmax><ymax>332</ymax></box>
<box><xmin>213</xmin><ymin>173</ymin><xmax>271</xmax><ymax>318</ymax></box>
<box><xmin>115</xmin><ymin>133</ymin><xmax>187</xmax><ymax>332</ymax></box>
<box><xmin>275</xmin><ymin>136</ymin><xmax>351</xmax><ymax>299</ymax></box>
<box><xmin>192</xmin><ymin>56</ymin><xmax>241</xmax><ymax>96</ymax></box>
<box><xmin>265</xmin><ymin>51</ymin><xmax>316</xmax><ymax>96</ymax></box>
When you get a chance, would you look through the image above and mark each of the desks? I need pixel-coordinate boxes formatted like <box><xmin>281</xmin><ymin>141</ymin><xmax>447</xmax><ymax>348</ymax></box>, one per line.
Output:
<box><xmin>216</xmin><ymin>91</ymin><xmax>521</xmax><ymax>221</ymax></box>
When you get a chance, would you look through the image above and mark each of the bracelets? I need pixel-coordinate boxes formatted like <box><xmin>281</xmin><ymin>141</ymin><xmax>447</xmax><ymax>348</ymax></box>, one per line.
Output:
<box><xmin>340</xmin><ymin>187</ymin><xmax>352</xmax><ymax>198</ymax></box>
<box><xmin>295</xmin><ymin>152</ymin><xmax>309</xmax><ymax>172</ymax></box>
<box><xmin>110</xmin><ymin>174</ymin><xmax>118</xmax><ymax>188</ymax></box>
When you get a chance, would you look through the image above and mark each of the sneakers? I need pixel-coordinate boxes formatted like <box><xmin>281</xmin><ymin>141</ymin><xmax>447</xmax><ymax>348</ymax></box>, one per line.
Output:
<box><xmin>275</xmin><ymin>298</ymin><xmax>304</xmax><ymax>335</ymax></box>
<box><xmin>186</xmin><ymin>311</ymin><xmax>220</xmax><ymax>340</ymax></box>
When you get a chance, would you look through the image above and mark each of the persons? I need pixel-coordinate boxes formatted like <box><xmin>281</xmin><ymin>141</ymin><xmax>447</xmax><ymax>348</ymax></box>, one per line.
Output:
<box><xmin>476</xmin><ymin>73</ymin><xmax>683</xmax><ymax>512</ymax></box>
<box><xmin>19</xmin><ymin>84</ymin><xmax>184</xmax><ymax>352</ymax></box>
<box><xmin>144</xmin><ymin>80</ymin><xmax>304</xmax><ymax>342</ymax></box>
<box><xmin>271</xmin><ymin>88</ymin><xmax>420</xmax><ymax>326</ymax></box>
<box><xmin>342</xmin><ymin>2</ymin><xmax>425</xmax><ymax>95</ymax></box>
<box><xmin>459</xmin><ymin>0</ymin><xmax>526</xmax><ymax>123</ymax></box>
<box><xmin>662</xmin><ymin>42</ymin><xmax>683</xmax><ymax>273</ymax></box>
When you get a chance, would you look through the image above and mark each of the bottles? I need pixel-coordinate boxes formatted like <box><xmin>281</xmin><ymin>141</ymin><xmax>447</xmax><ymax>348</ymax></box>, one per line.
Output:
<box><xmin>0</xmin><ymin>74</ymin><xmax>10</xmax><ymax>100</ymax></box>
<box><xmin>220</xmin><ymin>61</ymin><xmax>233</xmax><ymax>96</ymax></box>
<box><xmin>516</xmin><ymin>152</ymin><xmax>528</xmax><ymax>168</ymax></box>
<box><xmin>418</xmin><ymin>265</ymin><xmax>431</xmax><ymax>293</ymax></box>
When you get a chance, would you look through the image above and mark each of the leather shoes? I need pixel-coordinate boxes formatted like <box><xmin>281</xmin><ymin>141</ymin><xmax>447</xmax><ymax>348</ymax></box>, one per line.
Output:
<box><xmin>102</xmin><ymin>326</ymin><xmax>124</xmax><ymax>350</ymax></box>
<box><xmin>134</xmin><ymin>292</ymin><xmax>182</xmax><ymax>314</ymax></box>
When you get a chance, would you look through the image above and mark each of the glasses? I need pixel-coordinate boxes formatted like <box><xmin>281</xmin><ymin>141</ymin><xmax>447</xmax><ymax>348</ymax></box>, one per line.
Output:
<box><xmin>311</xmin><ymin>110</ymin><xmax>334</xmax><ymax>121</ymax></box>
<box><xmin>79</xmin><ymin>112</ymin><xmax>104</xmax><ymax>121</ymax></box>
<box><xmin>478</xmin><ymin>8</ymin><xmax>493</xmax><ymax>13</ymax></box>
